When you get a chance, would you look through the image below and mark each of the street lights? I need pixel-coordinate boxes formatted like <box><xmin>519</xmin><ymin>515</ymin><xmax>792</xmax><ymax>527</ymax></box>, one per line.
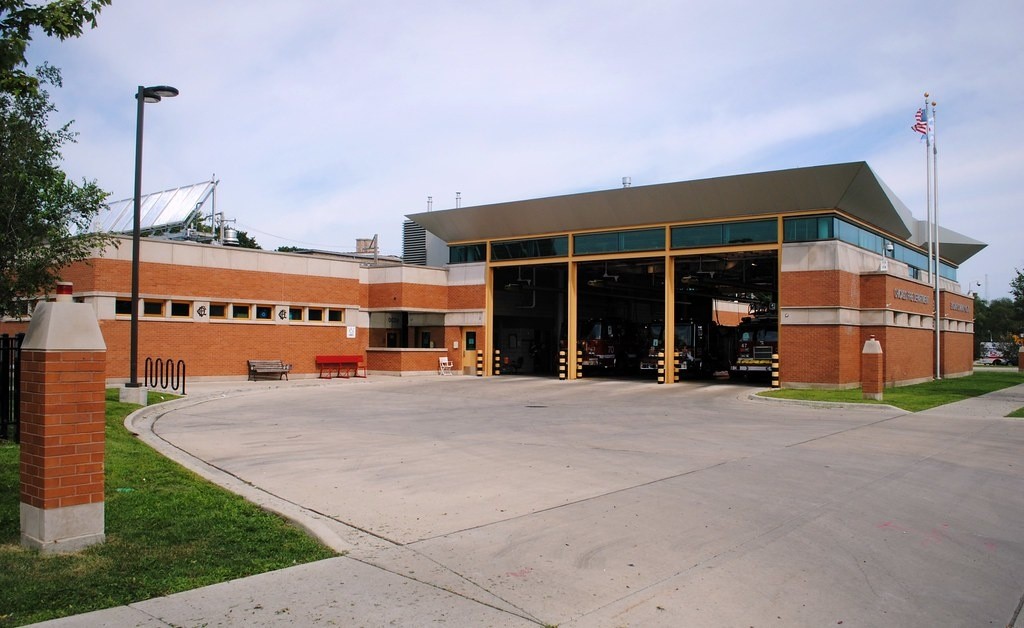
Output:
<box><xmin>124</xmin><ymin>83</ymin><xmax>180</xmax><ymax>387</ymax></box>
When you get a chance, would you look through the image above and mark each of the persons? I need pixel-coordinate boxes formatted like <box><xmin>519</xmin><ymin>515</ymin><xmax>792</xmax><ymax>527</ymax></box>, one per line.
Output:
<box><xmin>678</xmin><ymin>343</ymin><xmax>694</xmax><ymax>382</ymax></box>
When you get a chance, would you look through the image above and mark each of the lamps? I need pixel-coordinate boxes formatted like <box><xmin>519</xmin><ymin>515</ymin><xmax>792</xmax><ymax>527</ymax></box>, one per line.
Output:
<box><xmin>196</xmin><ymin>305</ymin><xmax>207</xmax><ymax>316</ymax></box>
<box><xmin>279</xmin><ymin>309</ymin><xmax>286</xmax><ymax>319</ymax></box>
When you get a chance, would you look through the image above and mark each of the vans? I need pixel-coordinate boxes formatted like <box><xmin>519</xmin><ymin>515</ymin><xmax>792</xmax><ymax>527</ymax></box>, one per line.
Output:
<box><xmin>980</xmin><ymin>342</ymin><xmax>1013</xmax><ymax>365</ymax></box>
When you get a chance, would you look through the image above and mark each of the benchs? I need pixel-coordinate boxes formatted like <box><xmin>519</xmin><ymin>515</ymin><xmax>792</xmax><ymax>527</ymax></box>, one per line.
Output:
<box><xmin>315</xmin><ymin>355</ymin><xmax>367</xmax><ymax>379</ymax></box>
<box><xmin>247</xmin><ymin>359</ymin><xmax>290</xmax><ymax>382</ymax></box>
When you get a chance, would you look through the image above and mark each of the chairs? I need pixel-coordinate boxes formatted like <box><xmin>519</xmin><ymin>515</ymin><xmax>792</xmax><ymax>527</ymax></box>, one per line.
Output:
<box><xmin>439</xmin><ymin>357</ymin><xmax>453</xmax><ymax>376</ymax></box>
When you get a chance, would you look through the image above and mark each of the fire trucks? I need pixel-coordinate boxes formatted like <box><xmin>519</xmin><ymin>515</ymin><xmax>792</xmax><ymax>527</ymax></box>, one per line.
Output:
<box><xmin>559</xmin><ymin>316</ymin><xmax>646</xmax><ymax>378</ymax></box>
<box><xmin>728</xmin><ymin>303</ymin><xmax>778</xmax><ymax>382</ymax></box>
<box><xmin>639</xmin><ymin>316</ymin><xmax>720</xmax><ymax>379</ymax></box>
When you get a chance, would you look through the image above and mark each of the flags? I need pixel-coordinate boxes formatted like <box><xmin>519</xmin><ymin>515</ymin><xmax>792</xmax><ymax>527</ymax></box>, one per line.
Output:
<box><xmin>911</xmin><ymin>108</ymin><xmax>934</xmax><ymax>141</ymax></box>
